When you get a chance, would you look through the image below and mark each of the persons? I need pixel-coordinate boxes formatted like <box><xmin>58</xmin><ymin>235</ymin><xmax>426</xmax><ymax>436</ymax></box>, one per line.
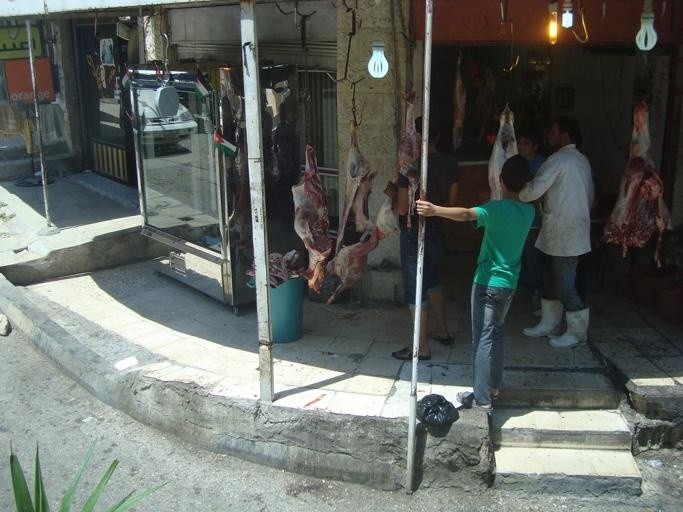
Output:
<box><xmin>414</xmin><ymin>154</ymin><xmax>537</xmax><ymax>413</ymax></box>
<box><xmin>509</xmin><ymin>125</ymin><xmax>544</xmax><ymax>321</ymax></box>
<box><xmin>388</xmin><ymin>116</ymin><xmax>460</xmax><ymax>363</ymax></box>
<box><xmin>516</xmin><ymin>116</ymin><xmax>596</xmax><ymax>350</ymax></box>
<box><xmin>444</xmin><ymin>83</ymin><xmax>489</xmax><ymax>152</ymax></box>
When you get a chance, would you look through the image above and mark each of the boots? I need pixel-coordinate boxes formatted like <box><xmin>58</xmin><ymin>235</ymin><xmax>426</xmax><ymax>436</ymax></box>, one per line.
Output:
<box><xmin>522</xmin><ymin>297</ymin><xmax>563</xmax><ymax>337</ymax></box>
<box><xmin>549</xmin><ymin>308</ymin><xmax>590</xmax><ymax>348</ymax></box>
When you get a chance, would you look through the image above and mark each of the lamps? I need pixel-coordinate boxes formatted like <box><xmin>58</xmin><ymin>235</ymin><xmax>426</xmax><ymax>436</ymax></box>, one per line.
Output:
<box><xmin>634</xmin><ymin>0</ymin><xmax>659</xmax><ymax>53</ymax></box>
<box><xmin>561</xmin><ymin>0</ymin><xmax>575</xmax><ymax>28</ymax></box>
<box><xmin>547</xmin><ymin>0</ymin><xmax>558</xmax><ymax>45</ymax></box>
<box><xmin>367</xmin><ymin>40</ymin><xmax>389</xmax><ymax>80</ymax></box>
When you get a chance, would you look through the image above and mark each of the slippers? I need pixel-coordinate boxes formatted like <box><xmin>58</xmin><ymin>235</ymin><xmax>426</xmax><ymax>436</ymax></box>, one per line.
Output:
<box><xmin>392</xmin><ymin>348</ymin><xmax>431</xmax><ymax>361</ymax></box>
<box><xmin>456</xmin><ymin>391</ymin><xmax>499</xmax><ymax>412</ymax></box>
<box><xmin>428</xmin><ymin>332</ymin><xmax>455</xmax><ymax>346</ymax></box>
